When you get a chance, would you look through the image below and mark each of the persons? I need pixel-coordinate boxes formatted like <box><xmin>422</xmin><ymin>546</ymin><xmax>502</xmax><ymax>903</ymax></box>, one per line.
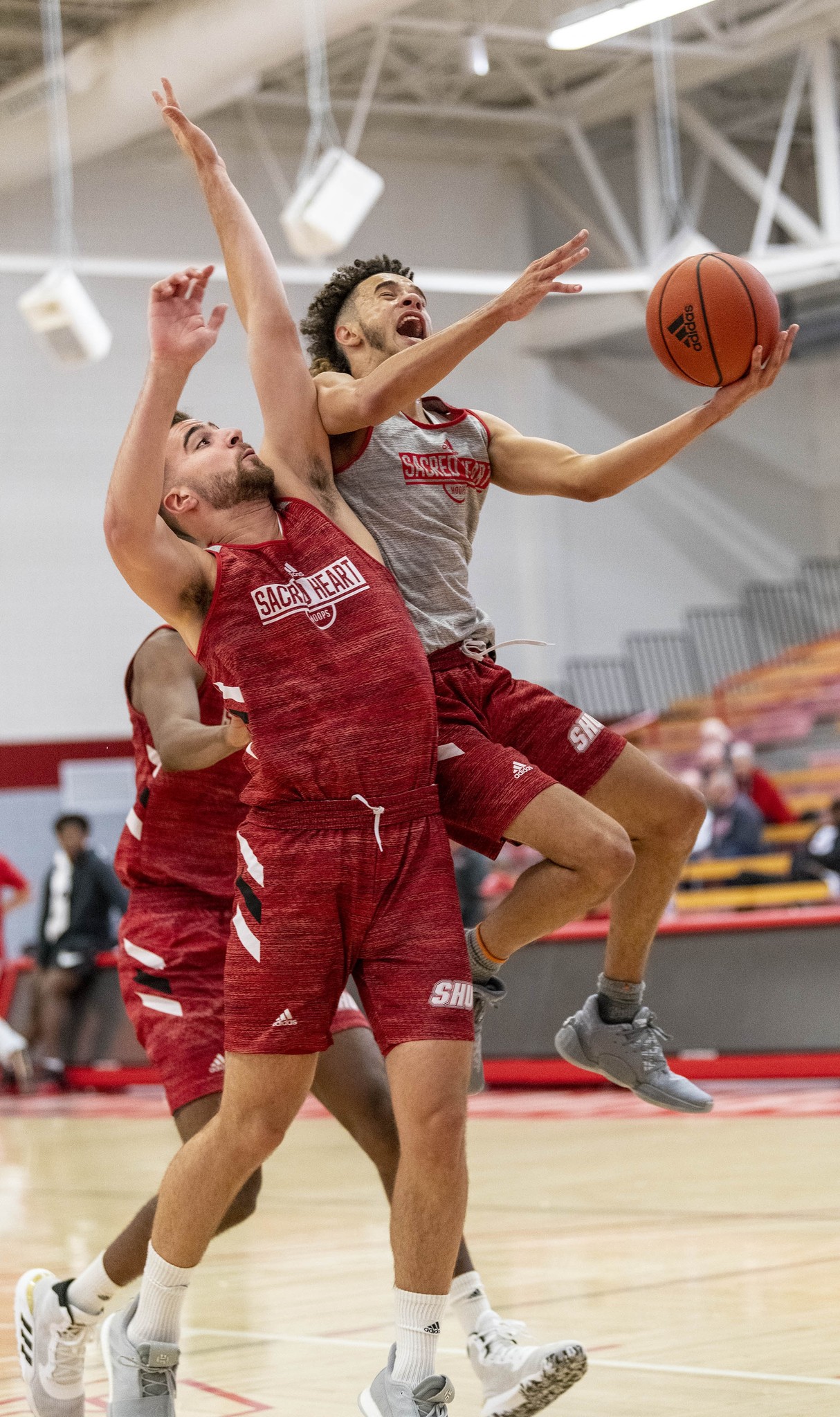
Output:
<box><xmin>447</xmin><ymin>718</ymin><xmax>840</xmax><ymax>930</ymax></box>
<box><xmin>15</xmin><ymin>622</ymin><xmax>589</xmax><ymax>1417</ymax></box>
<box><xmin>0</xmin><ymin>815</ymin><xmax>133</xmax><ymax>1087</ymax></box>
<box><xmin>298</xmin><ymin>230</ymin><xmax>797</xmax><ymax>1113</ymax></box>
<box><xmin>101</xmin><ymin>76</ymin><xmax>475</xmax><ymax>1417</ymax></box>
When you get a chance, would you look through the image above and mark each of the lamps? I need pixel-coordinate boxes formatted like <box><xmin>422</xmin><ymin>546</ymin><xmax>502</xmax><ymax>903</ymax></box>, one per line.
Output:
<box><xmin>13</xmin><ymin>271</ymin><xmax>111</xmax><ymax>367</ymax></box>
<box><xmin>279</xmin><ymin>151</ymin><xmax>384</xmax><ymax>253</ymax></box>
<box><xmin>544</xmin><ymin>0</ymin><xmax>713</xmax><ymax>52</ymax></box>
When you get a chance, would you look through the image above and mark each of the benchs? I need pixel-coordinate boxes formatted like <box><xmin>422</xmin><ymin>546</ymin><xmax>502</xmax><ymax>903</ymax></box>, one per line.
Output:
<box><xmin>480</xmin><ymin>626</ymin><xmax>840</xmax><ymax>909</ymax></box>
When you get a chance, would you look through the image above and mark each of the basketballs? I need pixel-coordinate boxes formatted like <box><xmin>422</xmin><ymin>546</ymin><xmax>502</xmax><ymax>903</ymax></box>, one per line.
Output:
<box><xmin>645</xmin><ymin>251</ymin><xmax>781</xmax><ymax>388</ymax></box>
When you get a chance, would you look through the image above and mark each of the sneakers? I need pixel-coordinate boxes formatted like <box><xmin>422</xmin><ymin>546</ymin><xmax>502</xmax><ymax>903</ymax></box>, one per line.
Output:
<box><xmin>464</xmin><ymin>1326</ymin><xmax>588</xmax><ymax>1417</ymax></box>
<box><xmin>552</xmin><ymin>993</ymin><xmax>713</xmax><ymax>1113</ymax></box>
<box><xmin>14</xmin><ymin>1269</ymin><xmax>101</xmax><ymax>1416</ymax></box>
<box><xmin>106</xmin><ymin>1296</ymin><xmax>180</xmax><ymax>1417</ymax></box>
<box><xmin>464</xmin><ymin>928</ymin><xmax>506</xmax><ymax>1093</ymax></box>
<box><xmin>359</xmin><ymin>1346</ymin><xmax>457</xmax><ymax>1417</ymax></box>
